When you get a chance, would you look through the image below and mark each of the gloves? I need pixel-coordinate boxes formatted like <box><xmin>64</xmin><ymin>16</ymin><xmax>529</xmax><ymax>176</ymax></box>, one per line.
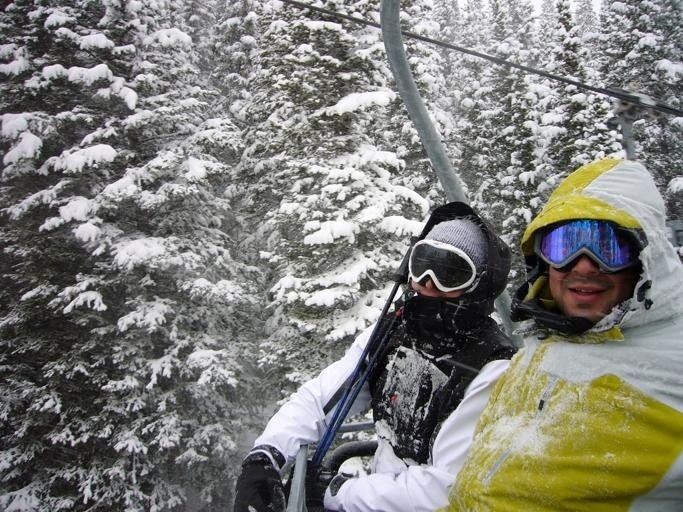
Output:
<box><xmin>233</xmin><ymin>445</ymin><xmax>286</xmax><ymax>511</ymax></box>
<box><xmin>286</xmin><ymin>462</ymin><xmax>337</xmax><ymax>506</ymax></box>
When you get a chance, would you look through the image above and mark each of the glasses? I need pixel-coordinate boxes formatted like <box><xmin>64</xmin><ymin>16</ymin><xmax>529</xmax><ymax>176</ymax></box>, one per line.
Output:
<box><xmin>408</xmin><ymin>240</ymin><xmax>477</xmax><ymax>294</ymax></box>
<box><xmin>533</xmin><ymin>217</ymin><xmax>641</xmax><ymax>273</ymax></box>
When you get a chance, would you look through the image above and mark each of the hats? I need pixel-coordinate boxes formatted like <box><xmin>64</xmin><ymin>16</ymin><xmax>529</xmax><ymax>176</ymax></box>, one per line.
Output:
<box><xmin>424</xmin><ymin>219</ymin><xmax>489</xmax><ymax>286</ymax></box>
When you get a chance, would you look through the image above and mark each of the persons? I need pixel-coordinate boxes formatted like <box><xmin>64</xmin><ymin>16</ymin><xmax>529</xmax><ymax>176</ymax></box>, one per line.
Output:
<box><xmin>230</xmin><ymin>200</ymin><xmax>519</xmax><ymax>512</ymax></box>
<box><xmin>435</xmin><ymin>156</ymin><xmax>683</xmax><ymax>512</ymax></box>
